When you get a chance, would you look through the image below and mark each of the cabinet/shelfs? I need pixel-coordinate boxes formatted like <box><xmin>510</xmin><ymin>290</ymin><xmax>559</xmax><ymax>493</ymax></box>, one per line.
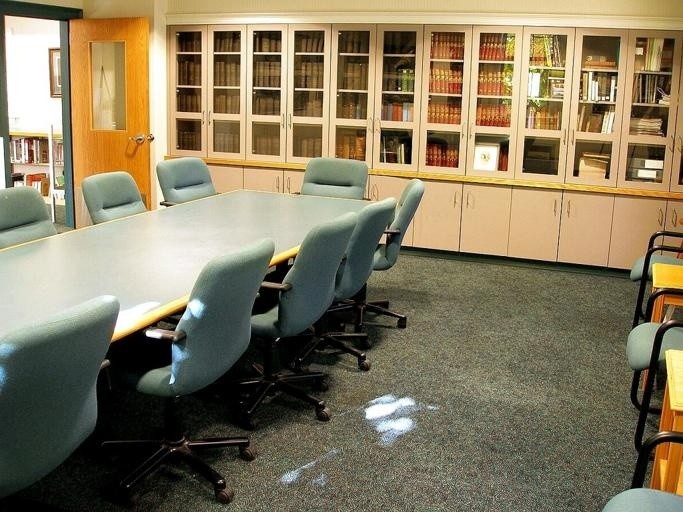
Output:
<box><xmin>508</xmin><ymin>185</ymin><xmax>616</xmax><ymax>277</ymax></box>
<box><xmin>209</xmin><ymin>164</ymin><xmax>243</xmax><ymax>194</ymax></box>
<box><xmin>364</xmin><ymin>177</ymin><xmax>413</xmax><ymax>262</ymax></box>
<box><xmin>170</xmin><ymin>25</ymin><xmax>682</xmax><ymax>191</ymax></box>
<box><xmin>608</xmin><ymin>190</ymin><xmax>683</xmax><ymax>278</ymax></box>
<box><xmin>9</xmin><ymin>131</ymin><xmax>65</xmax><ymax>207</ymax></box>
<box><xmin>413</xmin><ymin>178</ymin><xmax>512</xmax><ymax>267</ymax></box>
<box><xmin>244</xmin><ymin>167</ymin><xmax>306</xmax><ymax>195</ymax></box>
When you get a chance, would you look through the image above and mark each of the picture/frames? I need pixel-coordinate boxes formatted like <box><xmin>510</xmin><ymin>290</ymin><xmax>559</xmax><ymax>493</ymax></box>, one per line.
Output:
<box><xmin>48</xmin><ymin>47</ymin><xmax>61</xmax><ymax>99</ymax></box>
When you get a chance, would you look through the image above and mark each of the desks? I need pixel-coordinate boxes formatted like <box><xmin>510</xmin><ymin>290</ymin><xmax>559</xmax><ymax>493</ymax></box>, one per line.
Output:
<box><xmin>1</xmin><ymin>189</ymin><xmax>371</xmax><ymax>350</ymax></box>
<box><xmin>650</xmin><ymin>349</ymin><xmax>683</xmax><ymax>496</ymax></box>
<box><xmin>643</xmin><ymin>263</ymin><xmax>683</xmax><ymax>390</ymax></box>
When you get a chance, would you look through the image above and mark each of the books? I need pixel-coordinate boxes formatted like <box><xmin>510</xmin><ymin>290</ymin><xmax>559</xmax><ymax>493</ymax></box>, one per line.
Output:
<box><xmin>252</xmin><ymin>89</ymin><xmax>280</xmax><ymax>115</ymax></box>
<box><xmin>478</xmin><ymin>64</ymin><xmax>514</xmax><ymax>97</ymax></box>
<box><xmin>176</xmin><ymin>32</ymin><xmax>202</xmax><ymax>51</ymax></box>
<box><xmin>212</xmin><ymin>60</ymin><xmax>240</xmax><ymax>87</ymax></box>
<box><xmin>631</xmin><ymin>109</ymin><xmax>666</xmax><ymax>136</ymax></box>
<box><xmin>524</xmin><ymin>103</ymin><xmax>564</xmax><ymax>129</ymax></box>
<box><xmin>293</xmin><ymin>60</ymin><xmax>324</xmax><ymax>89</ymax></box>
<box><xmin>428</xmin><ymin>65</ymin><xmax>464</xmax><ymax>94</ymax></box>
<box><xmin>337</xmin><ymin>29</ymin><xmax>372</xmax><ymax>54</ymax></box>
<box><xmin>577</xmin><ymin>103</ymin><xmax>616</xmax><ymax>133</ymax></box>
<box><xmin>524</xmin><ymin>69</ymin><xmax>566</xmax><ymax>97</ymax></box>
<box><xmin>629</xmin><ymin>155</ymin><xmax>663</xmax><ymax>181</ymax></box>
<box><xmin>379</xmin><ymin>95</ymin><xmax>413</xmax><ymax>123</ymax></box>
<box><xmin>498</xmin><ymin>140</ymin><xmax>512</xmax><ymax>172</ymax></box>
<box><xmin>431</xmin><ymin>31</ymin><xmax>465</xmax><ymax>59</ymax></box>
<box><xmin>294</xmin><ymin>31</ymin><xmax>325</xmax><ymax>55</ymax></box>
<box><xmin>634</xmin><ymin>36</ymin><xmax>673</xmax><ymax>72</ymax></box>
<box><xmin>177</xmin><ymin>60</ymin><xmax>201</xmax><ymax>87</ymax></box>
<box><xmin>9</xmin><ymin>172</ymin><xmax>49</xmax><ymax>197</ymax></box>
<box><xmin>335</xmin><ymin>92</ymin><xmax>368</xmax><ymax>119</ymax></box>
<box><xmin>633</xmin><ymin>75</ymin><xmax>673</xmax><ymax>105</ymax></box>
<box><xmin>8</xmin><ymin>137</ymin><xmax>48</xmax><ymax>163</ymax></box>
<box><xmin>384</xmin><ymin>31</ymin><xmax>415</xmax><ymax>53</ymax></box>
<box><xmin>528</xmin><ymin>33</ymin><xmax>566</xmax><ymax>68</ymax></box>
<box><xmin>213</xmin><ymin>132</ymin><xmax>240</xmax><ymax>153</ymax></box>
<box><xmin>581</xmin><ymin>52</ymin><xmax>618</xmax><ymax>71</ymax></box>
<box><xmin>177</xmin><ymin>94</ymin><xmax>200</xmax><ymax>113</ymax></box>
<box><xmin>580</xmin><ymin>73</ymin><xmax>616</xmax><ymax>101</ymax></box>
<box><xmin>212</xmin><ymin>89</ymin><xmax>240</xmax><ymax>114</ymax></box>
<box><xmin>252</xmin><ymin>60</ymin><xmax>283</xmax><ymax>86</ymax></box>
<box><xmin>338</xmin><ymin>57</ymin><xmax>369</xmax><ymax>89</ymax></box>
<box><xmin>252</xmin><ymin>130</ymin><xmax>281</xmax><ymax>155</ymax></box>
<box><xmin>252</xmin><ymin>31</ymin><xmax>282</xmax><ymax>53</ymax></box>
<box><xmin>475</xmin><ymin>99</ymin><xmax>514</xmax><ymax>128</ymax></box>
<box><xmin>480</xmin><ymin>33</ymin><xmax>516</xmax><ymax>62</ymax></box>
<box><xmin>293</xmin><ymin>129</ymin><xmax>322</xmax><ymax>158</ymax></box>
<box><xmin>577</xmin><ymin>149</ymin><xmax>612</xmax><ymax>178</ymax></box>
<box><xmin>426</xmin><ymin>145</ymin><xmax>460</xmax><ymax>167</ymax></box>
<box><xmin>427</xmin><ymin>100</ymin><xmax>461</xmax><ymax>125</ymax></box>
<box><xmin>293</xmin><ymin>89</ymin><xmax>323</xmax><ymax>117</ymax></box>
<box><xmin>176</xmin><ymin>129</ymin><xmax>201</xmax><ymax>152</ymax></box>
<box><xmin>378</xmin><ymin>131</ymin><xmax>412</xmax><ymax>163</ymax></box>
<box><xmin>336</xmin><ymin>129</ymin><xmax>367</xmax><ymax>161</ymax></box>
<box><xmin>213</xmin><ymin>31</ymin><xmax>240</xmax><ymax>52</ymax></box>
<box><xmin>51</xmin><ymin>140</ymin><xmax>63</xmax><ymax>166</ymax></box>
<box><xmin>382</xmin><ymin>65</ymin><xmax>417</xmax><ymax>95</ymax></box>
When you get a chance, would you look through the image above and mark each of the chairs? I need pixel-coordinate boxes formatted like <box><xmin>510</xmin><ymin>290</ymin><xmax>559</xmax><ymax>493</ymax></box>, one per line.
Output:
<box><xmin>299</xmin><ymin>157</ymin><xmax>370</xmax><ymax>201</ymax></box>
<box><xmin>630</xmin><ymin>230</ymin><xmax>682</xmax><ymax>325</ymax></box>
<box><xmin>626</xmin><ymin>287</ymin><xmax>683</xmax><ymax>459</ymax></box>
<box><xmin>220</xmin><ymin>212</ymin><xmax>358</xmax><ymax>430</ymax></box>
<box><xmin>100</xmin><ymin>236</ymin><xmax>275</xmax><ymax>505</ymax></box>
<box><xmin>0</xmin><ymin>295</ymin><xmax>121</xmax><ymax>512</ymax></box>
<box><xmin>326</xmin><ymin>178</ymin><xmax>426</xmax><ymax>334</ymax></box>
<box><xmin>284</xmin><ymin>197</ymin><xmax>408</xmax><ymax>375</ymax></box>
<box><xmin>600</xmin><ymin>429</ymin><xmax>683</xmax><ymax>512</ymax></box>
<box><xmin>154</xmin><ymin>158</ymin><xmax>218</xmax><ymax>208</ymax></box>
<box><xmin>0</xmin><ymin>185</ymin><xmax>58</xmax><ymax>248</ymax></box>
<box><xmin>81</xmin><ymin>171</ymin><xmax>147</xmax><ymax>225</ymax></box>
